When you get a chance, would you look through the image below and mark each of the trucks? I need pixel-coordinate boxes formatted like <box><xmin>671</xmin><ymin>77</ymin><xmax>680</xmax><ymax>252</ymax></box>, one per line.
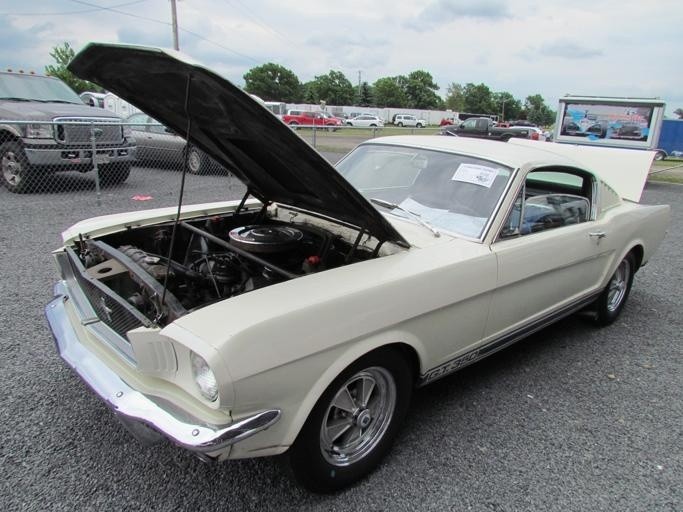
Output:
<box><xmin>654</xmin><ymin>118</ymin><xmax>683</xmax><ymax>160</ymax></box>
